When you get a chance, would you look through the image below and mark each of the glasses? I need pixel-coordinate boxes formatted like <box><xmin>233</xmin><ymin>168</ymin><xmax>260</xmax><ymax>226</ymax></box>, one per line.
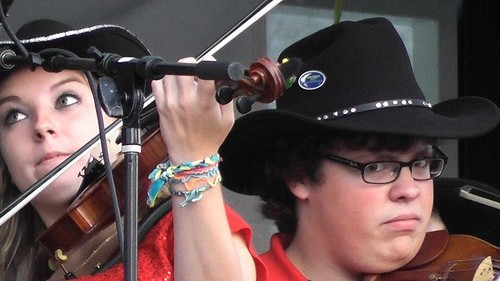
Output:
<box><xmin>324</xmin><ymin>146</ymin><xmax>448</xmax><ymax>184</ymax></box>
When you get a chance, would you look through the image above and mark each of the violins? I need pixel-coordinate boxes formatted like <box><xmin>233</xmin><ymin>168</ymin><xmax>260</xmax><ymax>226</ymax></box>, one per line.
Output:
<box><xmin>1</xmin><ymin>55</ymin><xmax>303</xmax><ymax>280</ymax></box>
<box><xmin>363</xmin><ymin>230</ymin><xmax>500</xmax><ymax>281</ymax></box>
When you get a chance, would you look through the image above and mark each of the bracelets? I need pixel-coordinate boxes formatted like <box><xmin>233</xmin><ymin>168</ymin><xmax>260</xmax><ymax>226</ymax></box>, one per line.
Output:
<box><xmin>146</xmin><ymin>153</ymin><xmax>222</xmax><ymax>207</ymax></box>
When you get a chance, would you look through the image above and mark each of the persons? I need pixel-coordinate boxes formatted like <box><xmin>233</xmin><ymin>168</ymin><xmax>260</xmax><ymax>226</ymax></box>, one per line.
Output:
<box><xmin>0</xmin><ymin>20</ymin><xmax>269</xmax><ymax>281</ymax></box>
<box><xmin>217</xmin><ymin>18</ymin><xmax>500</xmax><ymax>281</ymax></box>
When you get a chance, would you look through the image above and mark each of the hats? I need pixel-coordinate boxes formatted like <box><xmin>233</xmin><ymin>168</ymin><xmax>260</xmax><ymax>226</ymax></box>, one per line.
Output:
<box><xmin>0</xmin><ymin>19</ymin><xmax>152</xmax><ymax>98</ymax></box>
<box><xmin>217</xmin><ymin>17</ymin><xmax>500</xmax><ymax>195</ymax></box>
